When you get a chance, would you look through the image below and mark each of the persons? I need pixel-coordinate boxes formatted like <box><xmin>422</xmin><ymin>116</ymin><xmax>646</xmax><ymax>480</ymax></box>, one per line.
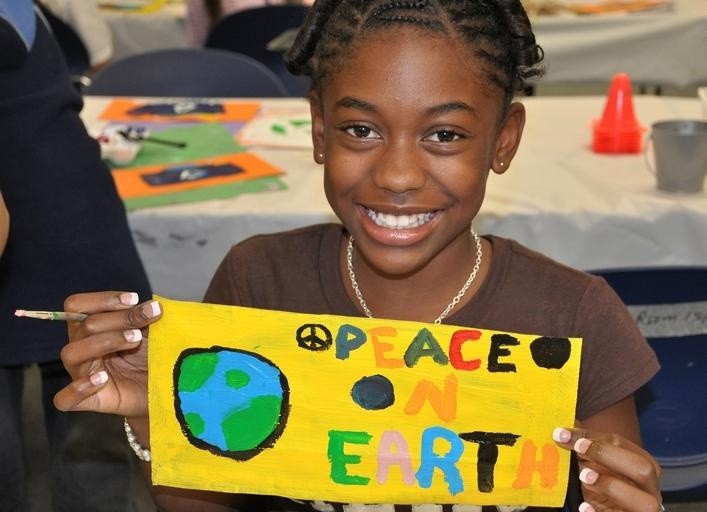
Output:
<box><xmin>0</xmin><ymin>0</ymin><xmax>155</xmax><ymax>510</ymax></box>
<box><xmin>51</xmin><ymin>2</ymin><xmax>672</xmax><ymax>511</ymax></box>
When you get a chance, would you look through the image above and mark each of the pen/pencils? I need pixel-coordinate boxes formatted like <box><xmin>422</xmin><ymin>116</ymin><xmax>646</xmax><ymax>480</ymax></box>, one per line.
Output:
<box><xmin>148</xmin><ymin>138</ymin><xmax>186</xmax><ymax>147</ymax></box>
<box><xmin>245</xmin><ymin>143</ymin><xmax>315</xmax><ymax>151</ymax></box>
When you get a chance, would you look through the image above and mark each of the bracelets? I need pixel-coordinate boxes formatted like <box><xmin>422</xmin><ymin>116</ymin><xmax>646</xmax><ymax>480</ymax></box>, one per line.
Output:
<box><xmin>124</xmin><ymin>413</ymin><xmax>152</xmax><ymax>472</ymax></box>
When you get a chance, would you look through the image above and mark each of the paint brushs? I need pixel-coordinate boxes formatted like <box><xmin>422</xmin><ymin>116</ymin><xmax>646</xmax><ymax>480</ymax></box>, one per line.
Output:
<box><xmin>14</xmin><ymin>310</ymin><xmax>88</xmax><ymax>322</ymax></box>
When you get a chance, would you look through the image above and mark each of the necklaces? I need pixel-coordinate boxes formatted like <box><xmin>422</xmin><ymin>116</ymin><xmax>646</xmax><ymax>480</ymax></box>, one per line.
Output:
<box><xmin>341</xmin><ymin>229</ymin><xmax>482</xmax><ymax>333</ymax></box>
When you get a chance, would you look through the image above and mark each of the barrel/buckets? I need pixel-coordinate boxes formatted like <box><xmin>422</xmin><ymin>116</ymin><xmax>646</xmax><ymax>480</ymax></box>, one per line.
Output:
<box><xmin>642</xmin><ymin>118</ymin><xmax>706</xmax><ymax>193</ymax></box>
